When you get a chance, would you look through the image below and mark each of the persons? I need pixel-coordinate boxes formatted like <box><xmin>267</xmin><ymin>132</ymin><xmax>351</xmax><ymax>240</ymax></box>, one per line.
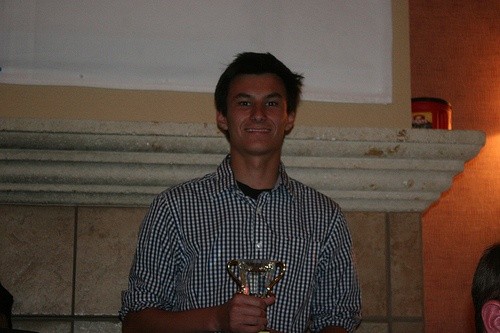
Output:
<box><xmin>471</xmin><ymin>243</ymin><xmax>500</xmax><ymax>333</ymax></box>
<box><xmin>119</xmin><ymin>52</ymin><xmax>364</xmax><ymax>332</ymax></box>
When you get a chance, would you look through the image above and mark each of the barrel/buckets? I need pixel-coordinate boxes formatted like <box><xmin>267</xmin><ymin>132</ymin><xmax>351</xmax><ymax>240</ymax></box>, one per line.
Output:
<box><xmin>411</xmin><ymin>96</ymin><xmax>451</xmax><ymax>130</ymax></box>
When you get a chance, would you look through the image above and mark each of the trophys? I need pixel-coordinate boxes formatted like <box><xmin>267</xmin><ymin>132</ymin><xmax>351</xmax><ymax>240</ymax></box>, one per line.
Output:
<box><xmin>226</xmin><ymin>257</ymin><xmax>286</xmax><ymax>303</ymax></box>
